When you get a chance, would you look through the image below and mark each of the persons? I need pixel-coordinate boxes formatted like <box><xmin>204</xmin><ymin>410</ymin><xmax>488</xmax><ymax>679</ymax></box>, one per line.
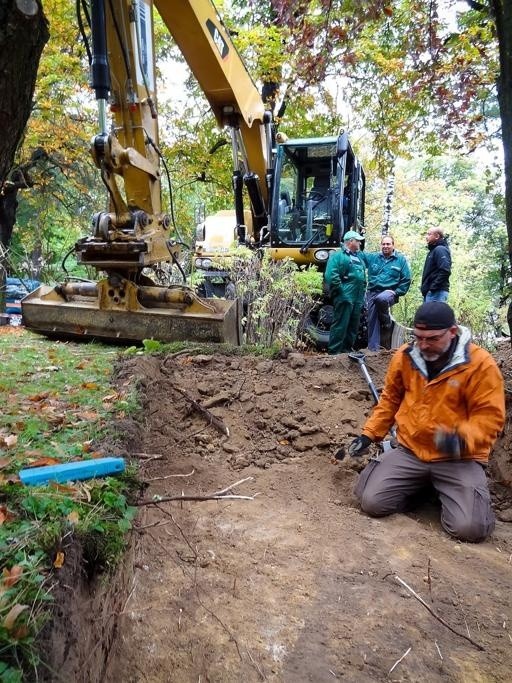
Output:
<box><xmin>353</xmin><ymin>234</ymin><xmax>411</xmax><ymax>350</ymax></box>
<box><xmin>348</xmin><ymin>299</ymin><xmax>507</xmax><ymax>544</ymax></box>
<box><xmin>419</xmin><ymin>226</ymin><xmax>453</xmax><ymax>303</ymax></box>
<box><xmin>324</xmin><ymin>229</ymin><xmax>366</xmax><ymax>355</ymax></box>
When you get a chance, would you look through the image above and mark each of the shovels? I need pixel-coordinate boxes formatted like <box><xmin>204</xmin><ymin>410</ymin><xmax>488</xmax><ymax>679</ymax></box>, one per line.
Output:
<box><xmin>348</xmin><ymin>353</ymin><xmax>399</xmax><ymax>453</ymax></box>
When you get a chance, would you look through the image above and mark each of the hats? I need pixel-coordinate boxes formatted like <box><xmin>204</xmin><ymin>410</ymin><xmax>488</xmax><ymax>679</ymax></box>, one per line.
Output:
<box><xmin>415</xmin><ymin>302</ymin><xmax>455</xmax><ymax>330</ymax></box>
<box><xmin>343</xmin><ymin>231</ymin><xmax>364</xmax><ymax>241</ymax></box>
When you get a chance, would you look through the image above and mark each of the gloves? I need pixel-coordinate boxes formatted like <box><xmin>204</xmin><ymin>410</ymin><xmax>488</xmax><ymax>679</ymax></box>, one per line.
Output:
<box><xmin>348</xmin><ymin>436</ymin><xmax>371</xmax><ymax>456</ymax></box>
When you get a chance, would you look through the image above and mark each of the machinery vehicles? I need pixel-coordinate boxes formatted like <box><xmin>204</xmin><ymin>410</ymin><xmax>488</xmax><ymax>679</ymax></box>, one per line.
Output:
<box><xmin>22</xmin><ymin>0</ymin><xmax>368</xmax><ymax>350</ymax></box>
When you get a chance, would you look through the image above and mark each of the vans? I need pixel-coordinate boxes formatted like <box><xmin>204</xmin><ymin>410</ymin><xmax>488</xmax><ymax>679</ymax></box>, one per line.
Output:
<box><xmin>5</xmin><ymin>276</ymin><xmax>43</xmax><ymax>313</ymax></box>
